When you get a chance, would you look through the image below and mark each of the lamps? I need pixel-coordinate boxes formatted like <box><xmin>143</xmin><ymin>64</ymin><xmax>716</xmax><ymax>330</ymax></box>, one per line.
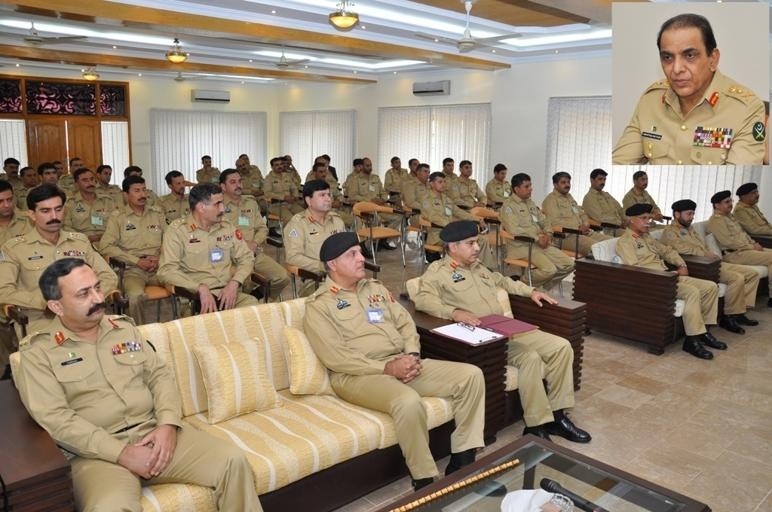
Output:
<box><xmin>77</xmin><ymin>60</ymin><xmax>102</xmax><ymax>84</ymax></box>
<box><xmin>325</xmin><ymin>1</ymin><xmax>362</xmax><ymax>34</ymax></box>
<box><xmin>165</xmin><ymin>36</ymin><xmax>191</xmax><ymax>65</ymax></box>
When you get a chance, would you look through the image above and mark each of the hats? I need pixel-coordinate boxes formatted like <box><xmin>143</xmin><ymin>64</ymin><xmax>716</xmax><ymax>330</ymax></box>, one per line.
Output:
<box><xmin>672</xmin><ymin>199</ymin><xmax>697</xmax><ymax>212</ymax></box>
<box><xmin>319</xmin><ymin>231</ymin><xmax>360</xmax><ymax>262</ymax></box>
<box><xmin>626</xmin><ymin>203</ymin><xmax>653</xmax><ymax>215</ymax></box>
<box><xmin>710</xmin><ymin>190</ymin><xmax>731</xmax><ymax>203</ymax></box>
<box><xmin>439</xmin><ymin>219</ymin><xmax>479</xmax><ymax>244</ymax></box>
<box><xmin>736</xmin><ymin>183</ymin><xmax>757</xmax><ymax>196</ymax></box>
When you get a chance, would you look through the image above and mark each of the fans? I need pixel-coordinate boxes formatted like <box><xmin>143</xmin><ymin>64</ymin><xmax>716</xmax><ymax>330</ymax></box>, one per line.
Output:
<box><xmin>261</xmin><ymin>45</ymin><xmax>309</xmax><ymax>74</ymax></box>
<box><xmin>429</xmin><ymin>3</ymin><xmax>524</xmax><ymax>56</ymax></box>
<box><xmin>10</xmin><ymin>21</ymin><xmax>90</xmax><ymax>48</ymax></box>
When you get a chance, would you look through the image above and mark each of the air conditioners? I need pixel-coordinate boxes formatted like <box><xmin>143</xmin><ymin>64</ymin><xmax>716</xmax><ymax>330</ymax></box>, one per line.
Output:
<box><xmin>411</xmin><ymin>80</ymin><xmax>451</xmax><ymax>97</ymax></box>
<box><xmin>190</xmin><ymin>89</ymin><xmax>231</xmax><ymax>104</ymax></box>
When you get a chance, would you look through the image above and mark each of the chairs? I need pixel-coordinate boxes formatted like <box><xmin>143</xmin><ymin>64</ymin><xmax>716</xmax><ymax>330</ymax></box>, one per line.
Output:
<box><xmin>547</xmin><ymin>217</ymin><xmax>595</xmax><ymax>268</ymax></box>
<box><xmin>107</xmin><ymin>248</ymin><xmax>180</xmax><ymax>324</ymax></box>
<box><xmin>351</xmin><ymin>199</ymin><xmax>409</xmax><ymax>271</ymax></box>
<box><xmin>104</xmin><ymin>245</ymin><xmax>177</xmax><ymax>320</ymax></box>
<box><xmin>415</xmin><ymin>211</ymin><xmax>445</xmax><ymax>265</ymax></box>
<box><xmin>498</xmin><ymin>225</ymin><xmax>556</xmax><ymax>291</ymax></box>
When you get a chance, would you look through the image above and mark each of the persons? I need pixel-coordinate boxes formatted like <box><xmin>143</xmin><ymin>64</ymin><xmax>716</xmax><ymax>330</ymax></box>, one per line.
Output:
<box><xmin>239</xmin><ymin>154</ymin><xmax>263</xmax><ymax>179</ymax></box>
<box><xmin>384</xmin><ymin>157</ymin><xmax>408</xmax><ymax>208</ymax></box>
<box><xmin>302</xmin><ymin>232</ymin><xmax>507</xmax><ymax>512</ymax></box>
<box><xmin>279</xmin><ymin>155</ymin><xmax>301</xmax><ymax>183</ymax></box>
<box><xmin>704</xmin><ymin>190</ymin><xmax>772</xmax><ymax>308</ymax></box>
<box><xmin>348</xmin><ymin>157</ymin><xmax>402</xmax><ymax>250</ymax></box>
<box><xmin>123</xmin><ymin>166</ymin><xmax>162</xmax><ymax>207</ymax></box>
<box><xmin>614</xmin><ymin>203</ymin><xmax>728</xmax><ymax>360</ymax></box>
<box><xmin>283</xmin><ymin>178</ymin><xmax>346</xmax><ymax>298</ymax></box>
<box><xmin>309</xmin><ymin>163</ymin><xmax>342</xmax><ymax>209</ymax></box>
<box><xmin>155</xmin><ymin>170</ymin><xmax>197</xmax><ymax>224</ymax></box>
<box><xmin>99</xmin><ymin>175</ymin><xmax>187</xmax><ymax>326</ymax></box>
<box><xmin>216</xmin><ymin>168</ymin><xmax>292</xmax><ymax>306</ymax></box>
<box><xmin>14</xmin><ymin>167</ymin><xmax>37</xmax><ymax>211</ymax></box>
<box><xmin>53</xmin><ymin>160</ymin><xmax>67</xmax><ymax>190</ymax></box>
<box><xmin>441</xmin><ymin>158</ymin><xmax>458</xmax><ymax>193</ymax></box>
<box><xmin>305</xmin><ymin>157</ymin><xmax>331</xmax><ymax>181</ymax></box>
<box><xmin>235</xmin><ymin>159</ymin><xmax>281</xmax><ymax>239</ymax></box>
<box><xmin>486</xmin><ymin>163</ymin><xmax>512</xmax><ymax>215</ymax></box>
<box><xmin>613</xmin><ymin>12</ymin><xmax>766</xmax><ymax>165</ymax></box>
<box><xmin>196</xmin><ymin>155</ymin><xmax>221</xmax><ymax>186</ymax></box>
<box><xmin>732</xmin><ymin>182</ymin><xmax>772</xmax><ymax>236</ymax></box>
<box><xmin>415</xmin><ymin>219</ymin><xmax>592</xmax><ymax>450</ymax></box>
<box><xmin>0</xmin><ymin>181</ymin><xmax>118</xmax><ymax>345</ymax></box>
<box><xmin>421</xmin><ymin>172</ymin><xmax>496</xmax><ymax>272</ymax></box>
<box><xmin>342</xmin><ymin>159</ymin><xmax>363</xmax><ymax>198</ymax></box>
<box><xmin>0</xmin><ymin>179</ymin><xmax>35</xmax><ymax>247</ymax></box>
<box><xmin>499</xmin><ymin>172</ymin><xmax>575</xmax><ymax>294</ymax></box>
<box><xmin>621</xmin><ymin>171</ymin><xmax>670</xmax><ymax>232</ymax></box>
<box><xmin>23</xmin><ymin>162</ymin><xmax>63</xmax><ymax>211</ymax></box>
<box><xmin>399</xmin><ymin>159</ymin><xmax>420</xmax><ymax>189</ymax></box>
<box><xmin>63</xmin><ymin>167</ymin><xmax>116</xmax><ymax>257</ymax></box>
<box><xmin>264</xmin><ymin>158</ymin><xmax>305</xmax><ymax>224</ymax></box>
<box><xmin>16</xmin><ymin>258</ymin><xmax>264</xmax><ymax>512</ymax></box>
<box><xmin>155</xmin><ymin>181</ymin><xmax>259</xmax><ymax>320</ymax></box>
<box><xmin>59</xmin><ymin>157</ymin><xmax>84</xmax><ymax>197</ymax></box>
<box><xmin>96</xmin><ymin>165</ymin><xmax>124</xmax><ymax>210</ymax></box>
<box><xmin>661</xmin><ymin>198</ymin><xmax>759</xmax><ymax>335</ymax></box>
<box><xmin>582</xmin><ymin>168</ymin><xmax>628</xmax><ymax>236</ymax></box>
<box><xmin>401</xmin><ymin>164</ymin><xmax>441</xmax><ymax>262</ymax></box>
<box><xmin>541</xmin><ymin>171</ymin><xmax>615</xmax><ymax>255</ymax></box>
<box><xmin>449</xmin><ymin>160</ymin><xmax>487</xmax><ymax>211</ymax></box>
<box><xmin>1</xmin><ymin>157</ymin><xmax>21</xmax><ymax>188</ymax></box>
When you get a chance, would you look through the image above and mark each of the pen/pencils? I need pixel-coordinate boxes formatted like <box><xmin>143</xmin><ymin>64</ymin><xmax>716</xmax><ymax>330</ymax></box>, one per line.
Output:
<box><xmin>475</xmin><ymin>322</ymin><xmax>494</xmax><ymax>332</ymax></box>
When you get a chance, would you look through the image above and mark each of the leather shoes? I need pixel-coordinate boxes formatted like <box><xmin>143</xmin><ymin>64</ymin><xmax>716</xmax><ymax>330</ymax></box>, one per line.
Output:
<box><xmin>359</xmin><ymin>243</ymin><xmax>373</xmax><ymax>258</ymax></box>
<box><xmin>547</xmin><ymin>416</ymin><xmax>592</xmax><ymax>444</ymax></box>
<box><xmin>722</xmin><ymin>317</ymin><xmax>747</xmax><ymax>334</ymax></box>
<box><xmin>378</xmin><ymin>240</ymin><xmax>398</xmax><ymax>252</ymax></box>
<box><xmin>523</xmin><ymin>425</ymin><xmax>572</xmax><ymax>470</ymax></box>
<box><xmin>445</xmin><ymin>453</ymin><xmax>507</xmax><ymax>498</ymax></box>
<box><xmin>410</xmin><ymin>479</ymin><xmax>445</xmax><ymax>512</ymax></box>
<box><xmin>682</xmin><ymin>338</ymin><xmax>714</xmax><ymax>361</ymax></box>
<box><xmin>701</xmin><ymin>332</ymin><xmax>727</xmax><ymax>349</ymax></box>
<box><xmin>738</xmin><ymin>313</ymin><xmax>760</xmax><ymax>326</ymax></box>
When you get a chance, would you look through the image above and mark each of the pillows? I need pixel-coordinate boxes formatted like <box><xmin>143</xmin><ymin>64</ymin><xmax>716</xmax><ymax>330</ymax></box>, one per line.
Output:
<box><xmin>189</xmin><ymin>337</ymin><xmax>279</xmax><ymax>422</ymax></box>
<box><xmin>278</xmin><ymin>329</ymin><xmax>341</xmax><ymax>396</ymax></box>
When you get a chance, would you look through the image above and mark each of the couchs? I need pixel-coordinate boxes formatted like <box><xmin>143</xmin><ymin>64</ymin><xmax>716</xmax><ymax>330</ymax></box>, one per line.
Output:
<box><xmin>397</xmin><ymin>276</ymin><xmax>587</xmax><ymax>433</ymax></box>
<box><xmin>690</xmin><ymin>217</ymin><xmax>768</xmax><ymax>292</ymax></box>
<box><xmin>578</xmin><ymin>240</ymin><xmax>723</xmax><ymax>347</ymax></box>
<box><xmin>0</xmin><ymin>295</ymin><xmax>459</xmax><ymax>512</ymax></box>
